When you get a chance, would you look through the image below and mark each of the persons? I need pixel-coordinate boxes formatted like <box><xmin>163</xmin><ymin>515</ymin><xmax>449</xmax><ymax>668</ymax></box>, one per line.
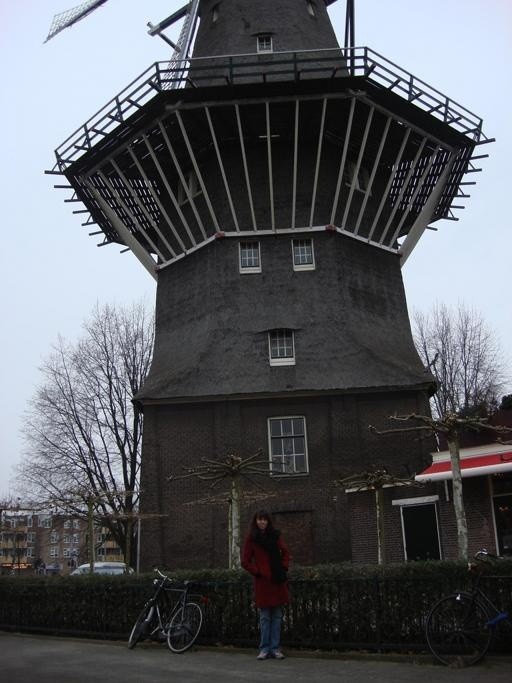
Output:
<box><xmin>241</xmin><ymin>507</ymin><xmax>293</xmax><ymax>660</ymax></box>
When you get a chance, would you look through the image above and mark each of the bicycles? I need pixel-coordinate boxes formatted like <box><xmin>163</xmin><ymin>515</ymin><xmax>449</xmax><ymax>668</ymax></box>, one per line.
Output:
<box><xmin>425</xmin><ymin>548</ymin><xmax>512</xmax><ymax>667</ymax></box>
<box><xmin>127</xmin><ymin>568</ymin><xmax>209</xmax><ymax>654</ymax></box>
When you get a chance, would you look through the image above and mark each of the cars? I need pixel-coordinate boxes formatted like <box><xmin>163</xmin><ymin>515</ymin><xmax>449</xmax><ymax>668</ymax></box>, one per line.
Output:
<box><xmin>70</xmin><ymin>561</ymin><xmax>135</xmax><ymax>575</ymax></box>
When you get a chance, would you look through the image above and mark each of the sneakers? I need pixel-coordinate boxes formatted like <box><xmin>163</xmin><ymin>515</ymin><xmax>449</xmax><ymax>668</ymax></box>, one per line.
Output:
<box><xmin>256</xmin><ymin>649</ymin><xmax>286</xmax><ymax>660</ymax></box>
